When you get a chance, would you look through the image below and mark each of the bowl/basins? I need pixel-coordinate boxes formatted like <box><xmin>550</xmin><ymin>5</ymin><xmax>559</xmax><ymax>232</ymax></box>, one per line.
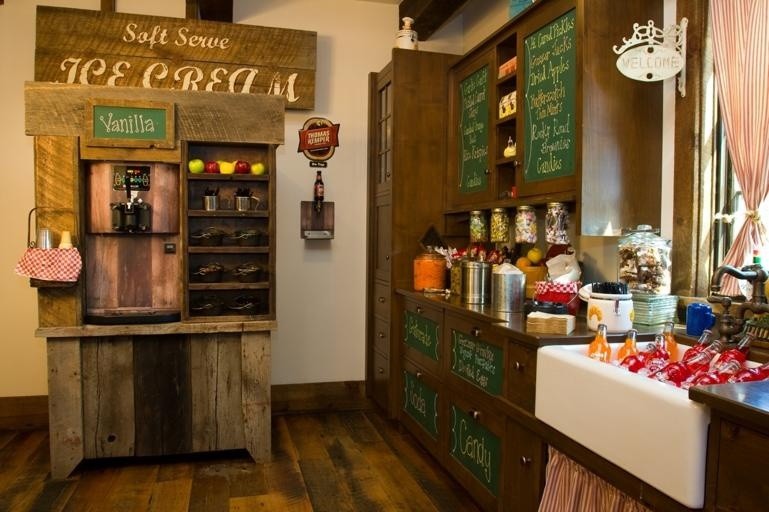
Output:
<box><xmin>523</xmin><ymin>301</ymin><xmax>567</xmax><ymax>321</ymax></box>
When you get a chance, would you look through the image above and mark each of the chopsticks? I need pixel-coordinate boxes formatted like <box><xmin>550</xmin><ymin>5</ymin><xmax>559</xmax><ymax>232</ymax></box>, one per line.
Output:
<box><xmin>235</xmin><ymin>187</ymin><xmax>254</xmax><ymax>197</ymax></box>
<box><xmin>591</xmin><ymin>281</ymin><xmax>628</xmax><ymax>295</ymax></box>
<box><xmin>204</xmin><ymin>186</ymin><xmax>220</xmax><ymax>196</ymax></box>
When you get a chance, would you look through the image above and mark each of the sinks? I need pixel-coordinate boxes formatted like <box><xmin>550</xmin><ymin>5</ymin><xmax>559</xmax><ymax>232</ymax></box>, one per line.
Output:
<box><xmin>532</xmin><ymin>338</ymin><xmax>769</xmax><ymax>510</ymax></box>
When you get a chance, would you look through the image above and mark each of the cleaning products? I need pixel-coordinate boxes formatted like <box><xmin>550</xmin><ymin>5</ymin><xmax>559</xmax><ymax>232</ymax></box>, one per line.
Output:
<box><xmin>392</xmin><ymin>16</ymin><xmax>418</xmax><ymax>50</ymax></box>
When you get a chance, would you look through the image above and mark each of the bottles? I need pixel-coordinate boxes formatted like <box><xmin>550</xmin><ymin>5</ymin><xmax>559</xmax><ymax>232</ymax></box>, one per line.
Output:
<box><xmin>544</xmin><ymin>202</ymin><xmax>571</xmax><ymax>245</ymax></box>
<box><xmin>586</xmin><ymin>324</ymin><xmax>612</xmax><ymax>365</ymax></box>
<box><xmin>470</xmin><ymin>210</ymin><xmax>488</xmax><ymax>243</ymax></box>
<box><xmin>490</xmin><ymin>207</ymin><xmax>510</xmax><ymax>244</ymax></box>
<box><xmin>514</xmin><ymin>205</ymin><xmax>538</xmax><ymax>243</ymax></box>
<box><xmin>618</xmin><ymin>223</ymin><xmax>674</xmax><ymax>300</ymax></box>
<box><xmin>617</xmin><ymin>321</ymin><xmax>768</xmax><ymax>388</ymax></box>
<box><xmin>313</xmin><ymin>170</ymin><xmax>325</xmax><ymax>201</ymax></box>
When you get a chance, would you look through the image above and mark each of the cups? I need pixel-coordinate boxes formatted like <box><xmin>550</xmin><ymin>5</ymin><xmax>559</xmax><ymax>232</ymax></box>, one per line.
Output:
<box><xmin>36</xmin><ymin>227</ymin><xmax>53</xmax><ymax>250</ymax></box>
<box><xmin>234</xmin><ymin>195</ymin><xmax>252</xmax><ymax>211</ymax></box>
<box><xmin>686</xmin><ymin>302</ymin><xmax>716</xmax><ymax>337</ymax></box>
<box><xmin>202</xmin><ymin>195</ymin><xmax>220</xmax><ymax>211</ymax></box>
<box><xmin>58</xmin><ymin>231</ymin><xmax>74</xmax><ymax>249</ymax></box>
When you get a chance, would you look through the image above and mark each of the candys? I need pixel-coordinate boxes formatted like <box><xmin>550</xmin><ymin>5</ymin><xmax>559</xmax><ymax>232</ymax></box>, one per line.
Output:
<box><xmin>545</xmin><ymin>208</ymin><xmax>570</xmax><ymax>245</ymax></box>
<box><xmin>470</xmin><ymin>216</ymin><xmax>489</xmax><ymax>242</ymax></box>
<box><xmin>491</xmin><ymin>213</ymin><xmax>510</xmax><ymax>243</ymax></box>
<box><xmin>515</xmin><ymin>211</ymin><xmax>537</xmax><ymax>243</ymax></box>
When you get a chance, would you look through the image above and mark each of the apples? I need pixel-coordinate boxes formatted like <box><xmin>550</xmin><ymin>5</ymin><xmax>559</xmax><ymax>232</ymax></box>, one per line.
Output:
<box><xmin>188</xmin><ymin>159</ymin><xmax>266</xmax><ymax>175</ymax></box>
<box><xmin>516</xmin><ymin>248</ymin><xmax>549</xmax><ymax>268</ymax></box>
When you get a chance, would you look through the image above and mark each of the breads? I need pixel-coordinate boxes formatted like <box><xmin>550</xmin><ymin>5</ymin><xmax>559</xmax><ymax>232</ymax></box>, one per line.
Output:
<box><xmin>526</xmin><ymin>311</ymin><xmax>576</xmax><ymax>336</ymax></box>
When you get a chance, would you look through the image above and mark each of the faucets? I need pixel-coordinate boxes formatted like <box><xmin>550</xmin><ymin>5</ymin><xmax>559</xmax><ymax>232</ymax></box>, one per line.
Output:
<box><xmin>710</xmin><ymin>263</ymin><xmax>769</xmax><ymax>312</ymax></box>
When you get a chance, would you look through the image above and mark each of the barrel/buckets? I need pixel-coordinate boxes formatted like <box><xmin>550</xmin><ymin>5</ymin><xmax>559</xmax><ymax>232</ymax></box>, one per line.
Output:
<box><xmin>461</xmin><ymin>261</ymin><xmax>490</xmax><ymax>305</ymax></box>
<box><xmin>491</xmin><ymin>273</ymin><xmax>526</xmax><ymax>312</ymax></box>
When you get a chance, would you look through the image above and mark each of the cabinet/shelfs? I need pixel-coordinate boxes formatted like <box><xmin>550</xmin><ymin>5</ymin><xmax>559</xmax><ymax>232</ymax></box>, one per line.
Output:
<box><xmin>686</xmin><ymin>376</ymin><xmax>767</xmax><ymax>512</ymax></box>
<box><xmin>392</xmin><ymin>286</ymin><xmax>672</xmax><ymax>511</ymax></box>
<box><xmin>444</xmin><ymin>0</ymin><xmax>666</xmax><ymax>239</ymax></box>
<box><xmin>22</xmin><ymin>78</ymin><xmax>287</xmax><ymax>485</ymax></box>
<box><xmin>363</xmin><ymin>47</ymin><xmax>463</xmax><ymax>425</ymax></box>
